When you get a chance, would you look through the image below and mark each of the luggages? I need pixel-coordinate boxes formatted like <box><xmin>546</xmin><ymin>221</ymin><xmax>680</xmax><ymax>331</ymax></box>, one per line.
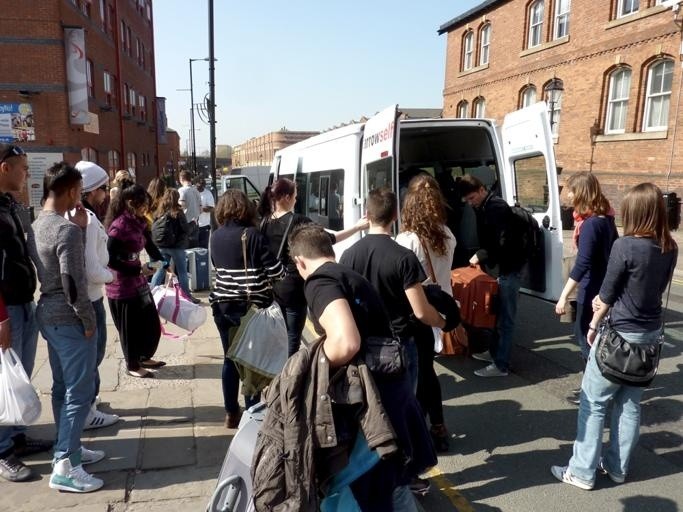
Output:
<box><xmin>188</xmin><ymin>248</ymin><xmax>209</xmax><ymax>291</ymax></box>
<box><xmin>450</xmin><ymin>260</ymin><xmax>500</xmax><ymax>331</ymax></box>
<box><xmin>202</xmin><ymin>398</ymin><xmax>273</xmax><ymax>512</ymax></box>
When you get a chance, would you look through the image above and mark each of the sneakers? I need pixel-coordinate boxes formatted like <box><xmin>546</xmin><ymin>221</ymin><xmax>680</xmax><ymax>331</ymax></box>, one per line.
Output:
<box><xmin>597</xmin><ymin>455</ymin><xmax>628</xmax><ymax>483</ymax></box>
<box><xmin>224</xmin><ymin>409</ymin><xmax>242</xmax><ymax>429</ymax></box>
<box><xmin>566</xmin><ymin>395</ymin><xmax>580</xmax><ymax>405</ymax></box>
<box><xmin>23</xmin><ymin>435</ymin><xmax>51</xmax><ymax>455</ymax></box>
<box><xmin>471</xmin><ymin>350</ymin><xmax>494</xmax><ymax>363</ymax></box>
<box><xmin>83</xmin><ymin>402</ymin><xmax>120</xmax><ymax>430</ymax></box>
<box><xmin>473</xmin><ymin>363</ymin><xmax>509</xmax><ymax>378</ymax></box>
<box><xmin>0</xmin><ymin>454</ymin><xmax>33</xmax><ymax>482</ymax></box>
<box><xmin>80</xmin><ymin>442</ymin><xmax>106</xmax><ymax>466</ymax></box>
<box><xmin>48</xmin><ymin>447</ymin><xmax>105</xmax><ymax>494</ymax></box>
<box><xmin>550</xmin><ymin>465</ymin><xmax>595</xmax><ymax>490</ymax></box>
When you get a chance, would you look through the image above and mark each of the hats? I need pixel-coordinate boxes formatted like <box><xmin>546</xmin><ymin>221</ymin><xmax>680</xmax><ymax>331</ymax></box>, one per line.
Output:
<box><xmin>74</xmin><ymin>160</ymin><xmax>110</xmax><ymax>194</ymax></box>
<box><xmin>0</xmin><ymin>142</ymin><xmax>27</xmax><ymax>163</ymax></box>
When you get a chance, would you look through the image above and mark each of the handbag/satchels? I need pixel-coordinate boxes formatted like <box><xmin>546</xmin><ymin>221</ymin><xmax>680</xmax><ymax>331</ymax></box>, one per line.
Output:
<box><xmin>360</xmin><ymin>337</ymin><xmax>402</xmax><ymax>377</ymax></box>
<box><xmin>0</xmin><ymin>347</ymin><xmax>42</xmax><ymax>427</ymax></box>
<box><xmin>150</xmin><ymin>279</ymin><xmax>208</xmax><ymax>339</ymax></box>
<box><xmin>593</xmin><ymin>317</ymin><xmax>667</xmax><ymax>388</ymax></box>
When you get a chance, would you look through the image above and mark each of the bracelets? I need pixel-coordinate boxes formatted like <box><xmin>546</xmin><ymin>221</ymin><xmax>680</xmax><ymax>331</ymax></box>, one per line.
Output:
<box><xmin>589</xmin><ymin>324</ymin><xmax>596</xmax><ymax>332</ymax></box>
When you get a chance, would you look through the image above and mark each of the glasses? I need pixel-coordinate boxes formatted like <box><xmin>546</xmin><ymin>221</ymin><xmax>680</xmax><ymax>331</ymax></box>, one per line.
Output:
<box><xmin>99</xmin><ymin>184</ymin><xmax>110</xmax><ymax>191</ymax></box>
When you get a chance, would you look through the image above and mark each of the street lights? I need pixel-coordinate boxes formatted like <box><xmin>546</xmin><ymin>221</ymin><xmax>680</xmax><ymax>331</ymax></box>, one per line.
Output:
<box><xmin>185</xmin><ymin>54</ymin><xmax>218</xmax><ymax>180</ymax></box>
<box><xmin>546</xmin><ymin>71</ymin><xmax>563</xmax><ymax>203</ymax></box>
<box><xmin>584</xmin><ymin>120</ymin><xmax>602</xmax><ymax>178</ymax></box>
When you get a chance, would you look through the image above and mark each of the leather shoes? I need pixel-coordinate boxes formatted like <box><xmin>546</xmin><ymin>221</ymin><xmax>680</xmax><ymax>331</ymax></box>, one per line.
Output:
<box><xmin>124</xmin><ymin>367</ymin><xmax>154</xmax><ymax>379</ymax></box>
<box><xmin>141</xmin><ymin>359</ymin><xmax>165</xmax><ymax>369</ymax></box>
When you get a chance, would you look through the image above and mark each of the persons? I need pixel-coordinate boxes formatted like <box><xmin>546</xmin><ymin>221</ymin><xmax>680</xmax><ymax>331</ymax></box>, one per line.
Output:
<box><xmin>456</xmin><ymin>173</ymin><xmax>522</xmax><ymax>377</ymax></box>
<box><xmin>553</xmin><ymin>171</ymin><xmax>619</xmax><ymax>403</ymax></box>
<box><xmin>549</xmin><ymin>181</ymin><xmax>679</xmax><ymax>491</ymax></box>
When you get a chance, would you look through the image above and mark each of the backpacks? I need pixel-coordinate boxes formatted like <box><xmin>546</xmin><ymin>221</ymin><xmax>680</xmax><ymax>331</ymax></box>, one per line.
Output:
<box><xmin>485</xmin><ymin>196</ymin><xmax>542</xmax><ymax>270</ymax></box>
